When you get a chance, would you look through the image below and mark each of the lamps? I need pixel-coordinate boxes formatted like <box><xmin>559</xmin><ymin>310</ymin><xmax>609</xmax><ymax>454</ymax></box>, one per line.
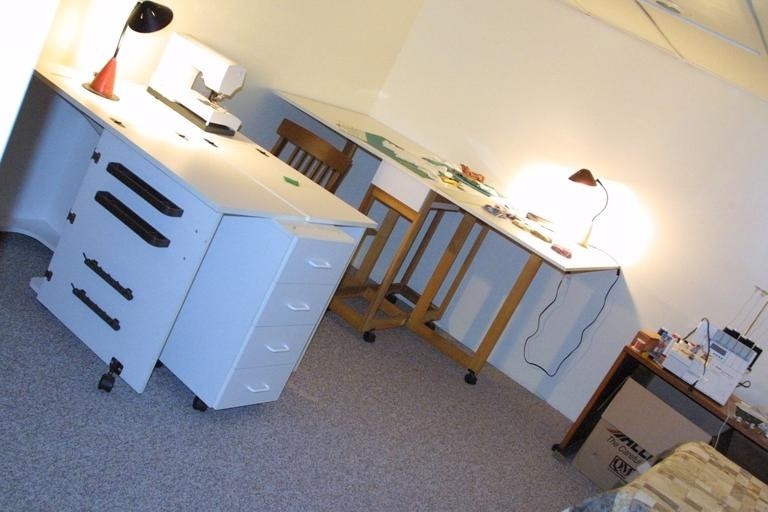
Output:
<box><xmin>81</xmin><ymin>1</ymin><xmax>174</xmax><ymax>105</ymax></box>
<box><xmin>565</xmin><ymin>170</ymin><xmax>611</xmax><ymax>249</ymax></box>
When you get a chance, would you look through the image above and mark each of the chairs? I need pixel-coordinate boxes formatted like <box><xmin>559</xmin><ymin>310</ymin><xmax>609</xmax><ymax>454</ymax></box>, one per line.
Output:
<box><xmin>269</xmin><ymin>117</ymin><xmax>354</xmax><ymax>196</ymax></box>
<box><xmin>271</xmin><ymin>88</ymin><xmax>620</xmax><ymax>384</ymax></box>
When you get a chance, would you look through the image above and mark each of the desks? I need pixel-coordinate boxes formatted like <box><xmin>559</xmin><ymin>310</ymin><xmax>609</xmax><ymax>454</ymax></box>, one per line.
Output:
<box><xmin>35</xmin><ymin>66</ymin><xmax>380</xmax><ymax>413</ymax></box>
<box><xmin>557</xmin><ymin>346</ymin><xmax>768</xmax><ymax>467</ymax></box>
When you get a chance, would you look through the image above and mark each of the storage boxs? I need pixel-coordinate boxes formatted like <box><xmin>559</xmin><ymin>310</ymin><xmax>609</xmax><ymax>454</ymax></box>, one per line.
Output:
<box><xmin>573</xmin><ymin>377</ymin><xmax>713</xmax><ymax>491</ymax></box>
